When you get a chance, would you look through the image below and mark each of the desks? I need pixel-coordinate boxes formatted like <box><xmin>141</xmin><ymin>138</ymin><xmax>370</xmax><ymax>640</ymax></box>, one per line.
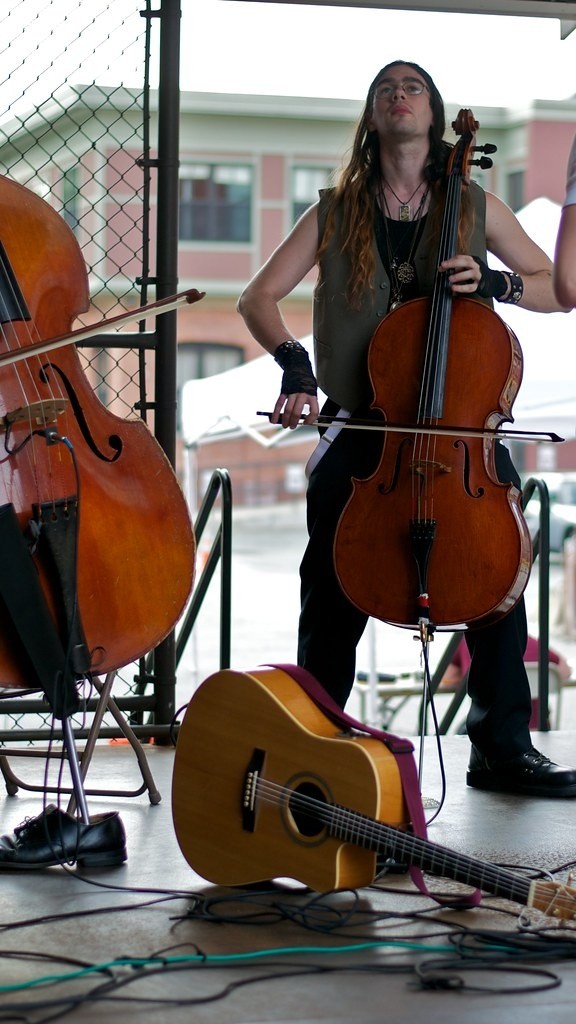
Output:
<box><xmin>354</xmin><ymin>678</ymin><xmax>576</xmax><ymax>735</ymax></box>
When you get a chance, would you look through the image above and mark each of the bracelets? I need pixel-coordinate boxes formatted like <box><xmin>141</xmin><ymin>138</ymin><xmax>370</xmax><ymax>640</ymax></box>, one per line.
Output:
<box><xmin>499</xmin><ymin>270</ymin><xmax>524</xmax><ymax>305</ymax></box>
<box><xmin>273</xmin><ymin>340</ymin><xmax>310</xmax><ymax>370</ymax></box>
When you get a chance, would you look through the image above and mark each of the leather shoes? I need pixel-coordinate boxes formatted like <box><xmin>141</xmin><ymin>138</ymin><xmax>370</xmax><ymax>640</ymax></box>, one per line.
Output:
<box><xmin>466</xmin><ymin>746</ymin><xmax>576</xmax><ymax>798</ymax></box>
<box><xmin>0</xmin><ymin>804</ymin><xmax>128</xmax><ymax>872</ymax></box>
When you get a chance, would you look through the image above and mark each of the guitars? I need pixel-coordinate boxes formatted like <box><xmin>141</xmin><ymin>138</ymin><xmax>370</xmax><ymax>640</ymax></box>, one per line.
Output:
<box><xmin>168</xmin><ymin>658</ymin><xmax>575</xmax><ymax>923</ymax></box>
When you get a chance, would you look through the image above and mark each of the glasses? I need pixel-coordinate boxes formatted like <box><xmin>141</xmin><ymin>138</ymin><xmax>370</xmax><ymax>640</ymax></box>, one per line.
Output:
<box><xmin>372</xmin><ymin>79</ymin><xmax>430</xmax><ymax>99</ymax></box>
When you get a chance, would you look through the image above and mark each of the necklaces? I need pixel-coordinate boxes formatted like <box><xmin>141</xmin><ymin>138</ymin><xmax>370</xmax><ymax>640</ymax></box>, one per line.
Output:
<box><xmin>379</xmin><ymin>169</ymin><xmax>431</xmax><ymax>312</ymax></box>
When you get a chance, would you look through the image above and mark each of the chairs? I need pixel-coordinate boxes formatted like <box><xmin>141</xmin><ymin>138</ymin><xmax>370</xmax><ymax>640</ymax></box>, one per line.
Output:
<box><xmin>0</xmin><ymin>670</ymin><xmax>165</xmax><ymax>817</ymax></box>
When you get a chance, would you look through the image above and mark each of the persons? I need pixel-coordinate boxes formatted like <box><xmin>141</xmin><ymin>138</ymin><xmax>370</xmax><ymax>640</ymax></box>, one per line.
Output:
<box><xmin>443</xmin><ymin>634</ymin><xmax>571</xmax><ymax>734</ymax></box>
<box><xmin>553</xmin><ymin>134</ymin><xmax>576</xmax><ymax>309</ymax></box>
<box><xmin>235</xmin><ymin>60</ymin><xmax>576</xmax><ymax>794</ymax></box>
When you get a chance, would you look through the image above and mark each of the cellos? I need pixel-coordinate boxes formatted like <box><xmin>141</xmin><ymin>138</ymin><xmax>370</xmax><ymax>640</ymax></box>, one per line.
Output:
<box><xmin>328</xmin><ymin>104</ymin><xmax>535</xmax><ymax>789</ymax></box>
<box><xmin>0</xmin><ymin>172</ymin><xmax>197</xmax><ymax>826</ymax></box>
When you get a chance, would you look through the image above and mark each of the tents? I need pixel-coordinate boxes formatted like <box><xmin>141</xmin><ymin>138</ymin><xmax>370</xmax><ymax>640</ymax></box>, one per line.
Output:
<box><xmin>179</xmin><ymin>195</ymin><xmax>576</xmax><ymax>521</ymax></box>
